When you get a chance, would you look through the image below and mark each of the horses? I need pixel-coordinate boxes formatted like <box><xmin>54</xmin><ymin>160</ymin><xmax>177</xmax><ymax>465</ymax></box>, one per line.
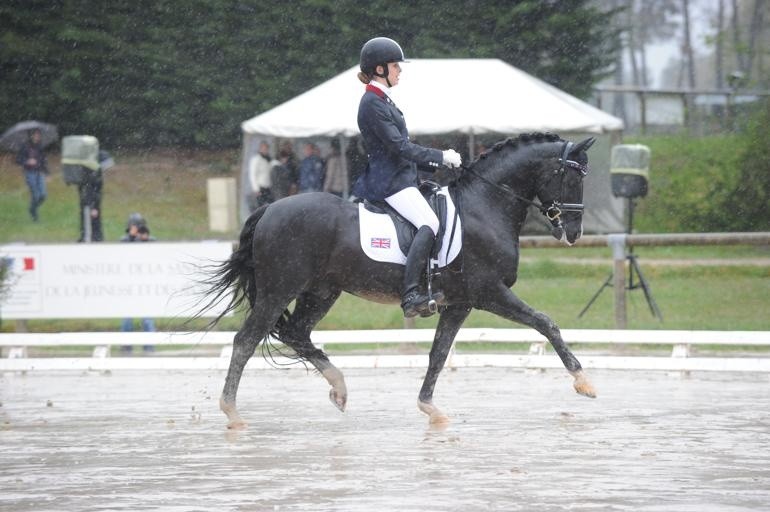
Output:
<box><xmin>165</xmin><ymin>131</ymin><xmax>597</xmax><ymax>422</ymax></box>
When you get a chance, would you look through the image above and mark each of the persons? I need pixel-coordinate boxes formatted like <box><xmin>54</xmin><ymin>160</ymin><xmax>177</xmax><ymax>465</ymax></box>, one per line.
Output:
<box><xmin>248</xmin><ymin>135</ymin><xmax>355</xmax><ymax>208</ymax></box>
<box><xmin>15</xmin><ymin>127</ymin><xmax>52</xmax><ymax>221</ymax></box>
<box><xmin>355</xmin><ymin>38</ymin><xmax>461</xmax><ymax>317</ymax></box>
<box><xmin>79</xmin><ymin>165</ymin><xmax>104</xmax><ymax>243</ymax></box>
<box><xmin>135</xmin><ymin>227</ymin><xmax>156</xmax><ymax>242</ymax></box>
<box><xmin>121</xmin><ymin>222</ymin><xmax>157</xmax><ymax>352</ymax></box>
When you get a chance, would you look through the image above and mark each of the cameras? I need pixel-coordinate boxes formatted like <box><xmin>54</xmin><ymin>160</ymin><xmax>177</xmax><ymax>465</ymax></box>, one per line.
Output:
<box><xmin>138</xmin><ymin>226</ymin><xmax>150</xmax><ymax>237</ymax></box>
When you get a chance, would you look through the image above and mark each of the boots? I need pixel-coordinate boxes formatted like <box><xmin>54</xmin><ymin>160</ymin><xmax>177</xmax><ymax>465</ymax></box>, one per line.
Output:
<box><xmin>400</xmin><ymin>225</ymin><xmax>445</xmax><ymax>318</ymax></box>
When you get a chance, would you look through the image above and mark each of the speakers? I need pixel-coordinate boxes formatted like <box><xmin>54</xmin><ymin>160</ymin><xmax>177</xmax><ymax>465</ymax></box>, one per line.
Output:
<box><xmin>60</xmin><ymin>134</ymin><xmax>100</xmax><ymax>186</ymax></box>
<box><xmin>610</xmin><ymin>144</ymin><xmax>651</xmax><ymax>198</ymax></box>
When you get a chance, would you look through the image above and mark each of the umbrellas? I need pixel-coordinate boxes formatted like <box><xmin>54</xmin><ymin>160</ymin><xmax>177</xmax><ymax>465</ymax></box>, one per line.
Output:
<box><xmin>1</xmin><ymin>121</ymin><xmax>61</xmax><ymax>148</ymax></box>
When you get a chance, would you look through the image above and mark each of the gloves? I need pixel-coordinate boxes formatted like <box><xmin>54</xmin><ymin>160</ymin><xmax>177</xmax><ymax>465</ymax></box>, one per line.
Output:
<box><xmin>442</xmin><ymin>149</ymin><xmax>461</xmax><ymax>169</ymax></box>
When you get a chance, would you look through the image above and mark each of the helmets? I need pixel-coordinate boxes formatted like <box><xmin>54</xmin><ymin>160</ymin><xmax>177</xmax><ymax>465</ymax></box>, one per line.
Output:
<box><xmin>360</xmin><ymin>37</ymin><xmax>404</xmax><ymax>72</ymax></box>
<box><xmin>129</xmin><ymin>213</ymin><xmax>145</xmax><ymax>224</ymax></box>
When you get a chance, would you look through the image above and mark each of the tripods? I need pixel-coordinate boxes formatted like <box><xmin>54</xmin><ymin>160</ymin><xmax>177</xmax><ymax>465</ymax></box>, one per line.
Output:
<box><xmin>578</xmin><ymin>198</ymin><xmax>665</xmax><ymax>322</ymax></box>
